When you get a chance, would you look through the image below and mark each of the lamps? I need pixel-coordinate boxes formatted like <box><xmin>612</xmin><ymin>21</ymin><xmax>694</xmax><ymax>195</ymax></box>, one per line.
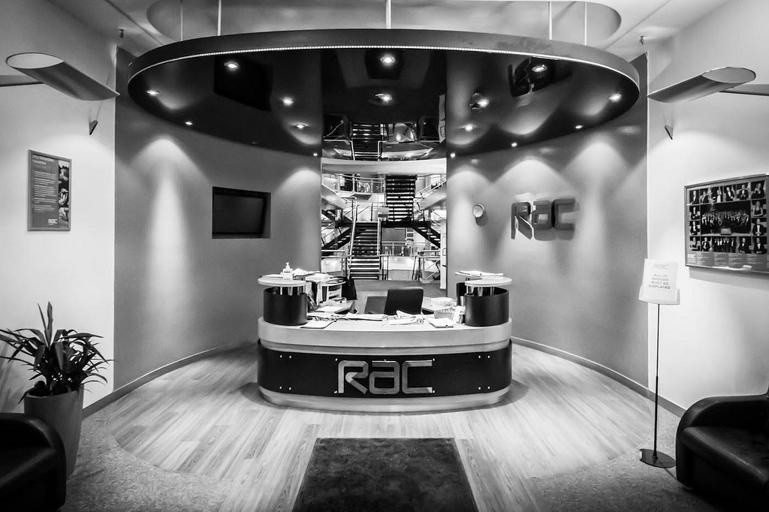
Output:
<box><xmin>0</xmin><ymin>52</ymin><xmax>120</xmax><ymax>101</ymax></box>
<box><xmin>647</xmin><ymin>67</ymin><xmax>768</xmax><ymax>103</ymax></box>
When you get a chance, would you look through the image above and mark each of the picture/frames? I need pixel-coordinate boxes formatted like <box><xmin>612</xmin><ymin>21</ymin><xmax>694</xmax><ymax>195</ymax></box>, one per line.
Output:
<box><xmin>684</xmin><ymin>172</ymin><xmax>769</xmax><ymax>274</ymax></box>
<box><xmin>27</xmin><ymin>150</ymin><xmax>72</xmax><ymax>231</ymax></box>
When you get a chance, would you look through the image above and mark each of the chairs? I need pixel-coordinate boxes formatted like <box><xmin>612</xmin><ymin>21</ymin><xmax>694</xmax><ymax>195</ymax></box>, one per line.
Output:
<box><xmin>1</xmin><ymin>413</ymin><xmax>66</xmax><ymax>512</ymax></box>
<box><xmin>676</xmin><ymin>387</ymin><xmax>768</xmax><ymax>511</ymax></box>
<box><xmin>368</xmin><ymin>289</ymin><xmax>423</xmax><ymax>315</ymax></box>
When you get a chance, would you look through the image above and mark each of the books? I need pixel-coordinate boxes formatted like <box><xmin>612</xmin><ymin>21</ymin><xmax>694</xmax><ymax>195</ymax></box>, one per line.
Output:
<box><xmin>430</xmin><ymin>318</ymin><xmax>454</xmax><ymax>328</ymax></box>
<box><xmin>455</xmin><ymin>269</ymin><xmax>503</xmax><ymax>279</ymax></box>
<box><xmin>301</xmin><ymin>320</ymin><xmax>332</xmax><ymax>328</ymax></box>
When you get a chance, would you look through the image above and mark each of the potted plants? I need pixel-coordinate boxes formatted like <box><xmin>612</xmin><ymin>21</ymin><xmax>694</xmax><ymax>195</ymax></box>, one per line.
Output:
<box><xmin>0</xmin><ymin>302</ymin><xmax>117</xmax><ymax>476</ymax></box>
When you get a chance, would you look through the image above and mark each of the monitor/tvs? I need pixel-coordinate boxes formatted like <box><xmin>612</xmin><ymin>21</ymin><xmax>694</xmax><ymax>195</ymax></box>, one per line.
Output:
<box><xmin>212</xmin><ymin>185</ymin><xmax>266</xmax><ymax>234</ymax></box>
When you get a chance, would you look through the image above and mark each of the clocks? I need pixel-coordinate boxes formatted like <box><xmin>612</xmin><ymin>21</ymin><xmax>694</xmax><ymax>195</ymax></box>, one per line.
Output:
<box><xmin>472</xmin><ymin>204</ymin><xmax>484</xmax><ymax>218</ymax></box>
